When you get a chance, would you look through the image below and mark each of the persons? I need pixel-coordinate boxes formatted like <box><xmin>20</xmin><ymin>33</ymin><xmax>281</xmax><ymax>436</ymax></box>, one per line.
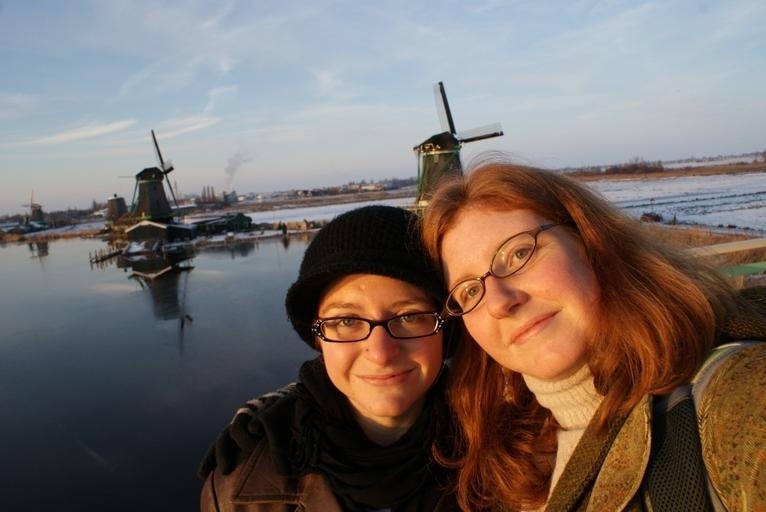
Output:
<box><xmin>199</xmin><ymin>205</ymin><xmax>537</xmax><ymax>507</ymax></box>
<box><xmin>201</xmin><ymin>159</ymin><xmax>766</xmax><ymax>512</ymax></box>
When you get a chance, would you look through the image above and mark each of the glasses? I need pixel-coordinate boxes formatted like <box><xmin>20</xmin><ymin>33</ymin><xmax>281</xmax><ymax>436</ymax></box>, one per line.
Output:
<box><xmin>305</xmin><ymin>310</ymin><xmax>446</xmax><ymax>343</ymax></box>
<box><xmin>437</xmin><ymin>221</ymin><xmax>567</xmax><ymax>321</ymax></box>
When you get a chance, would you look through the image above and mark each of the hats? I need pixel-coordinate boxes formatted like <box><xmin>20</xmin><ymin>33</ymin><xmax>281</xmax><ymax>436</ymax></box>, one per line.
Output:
<box><xmin>285</xmin><ymin>204</ymin><xmax>454</xmax><ymax>353</ymax></box>
